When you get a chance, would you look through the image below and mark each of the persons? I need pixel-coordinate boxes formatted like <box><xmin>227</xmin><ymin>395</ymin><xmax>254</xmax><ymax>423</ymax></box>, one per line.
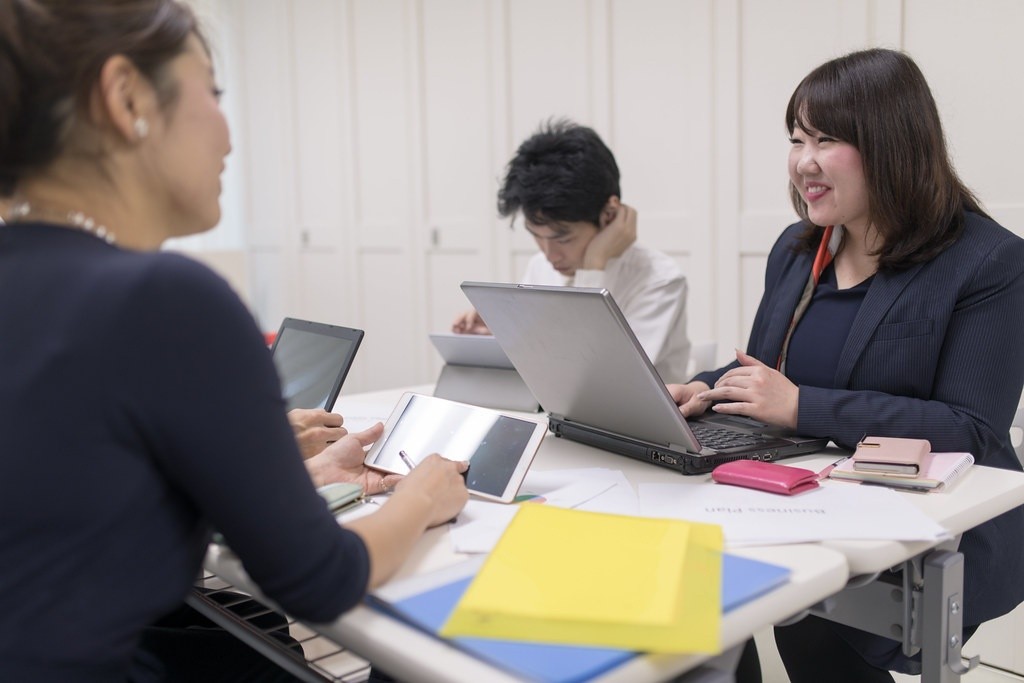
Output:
<box><xmin>154</xmin><ymin>408</ymin><xmax>349</xmax><ymax>667</ymax></box>
<box><xmin>667</xmin><ymin>49</ymin><xmax>1023</xmax><ymax>683</ymax></box>
<box><xmin>0</xmin><ymin>0</ymin><xmax>470</xmax><ymax>683</ymax></box>
<box><xmin>452</xmin><ymin>115</ymin><xmax>692</xmax><ymax>385</ymax></box>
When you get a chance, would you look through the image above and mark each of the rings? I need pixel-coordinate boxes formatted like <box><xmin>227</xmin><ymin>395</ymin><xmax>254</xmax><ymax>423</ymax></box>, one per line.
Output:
<box><xmin>381</xmin><ymin>479</ymin><xmax>388</xmax><ymax>492</ymax></box>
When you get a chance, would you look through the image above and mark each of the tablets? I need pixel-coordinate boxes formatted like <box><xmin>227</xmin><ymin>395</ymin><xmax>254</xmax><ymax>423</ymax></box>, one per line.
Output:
<box><xmin>269</xmin><ymin>317</ymin><xmax>364</xmax><ymax>413</ymax></box>
<box><xmin>364</xmin><ymin>392</ymin><xmax>548</xmax><ymax>505</ymax></box>
<box><xmin>427</xmin><ymin>334</ymin><xmax>513</xmax><ymax>369</ymax></box>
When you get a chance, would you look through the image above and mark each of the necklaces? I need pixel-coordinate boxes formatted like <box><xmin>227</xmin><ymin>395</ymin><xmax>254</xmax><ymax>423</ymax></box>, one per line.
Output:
<box><xmin>0</xmin><ymin>204</ymin><xmax>115</xmax><ymax>244</ymax></box>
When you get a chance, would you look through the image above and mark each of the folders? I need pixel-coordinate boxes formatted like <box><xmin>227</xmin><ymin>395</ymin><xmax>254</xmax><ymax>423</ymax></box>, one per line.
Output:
<box><xmin>386</xmin><ymin>550</ymin><xmax>791</xmax><ymax>683</ymax></box>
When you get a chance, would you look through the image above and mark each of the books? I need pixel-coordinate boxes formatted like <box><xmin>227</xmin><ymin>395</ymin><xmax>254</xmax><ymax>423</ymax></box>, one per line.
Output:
<box><xmin>829</xmin><ymin>452</ymin><xmax>975</xmax><ymax>494</ymax></box>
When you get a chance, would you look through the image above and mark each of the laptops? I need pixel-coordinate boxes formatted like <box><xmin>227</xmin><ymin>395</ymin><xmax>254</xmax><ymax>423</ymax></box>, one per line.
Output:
<box><xmin>459</xmin><ymin>281</ymin><xmax>830</xmax><ymax>475</ymax></box>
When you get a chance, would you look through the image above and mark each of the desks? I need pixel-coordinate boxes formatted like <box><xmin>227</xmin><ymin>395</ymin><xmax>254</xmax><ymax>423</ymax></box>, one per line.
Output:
<box><xmin>184</xmin><ymin>379</ymin><xmax>1024</xmax><ymax>683</ymax></box>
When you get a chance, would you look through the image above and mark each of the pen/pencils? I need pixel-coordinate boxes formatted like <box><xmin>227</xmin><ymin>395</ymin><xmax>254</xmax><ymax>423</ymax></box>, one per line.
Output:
<box><xmin>399</xmin><ymin>450</ymin><xmax>456</xmax><ymax>523</ymax></box>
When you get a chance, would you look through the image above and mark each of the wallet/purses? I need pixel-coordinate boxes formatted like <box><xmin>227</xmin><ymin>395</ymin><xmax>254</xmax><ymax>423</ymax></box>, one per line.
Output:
<box><xmin>711</xmin><ymin>460</ymin><xmax>820</xmax><ymax>496</ymax></box>
